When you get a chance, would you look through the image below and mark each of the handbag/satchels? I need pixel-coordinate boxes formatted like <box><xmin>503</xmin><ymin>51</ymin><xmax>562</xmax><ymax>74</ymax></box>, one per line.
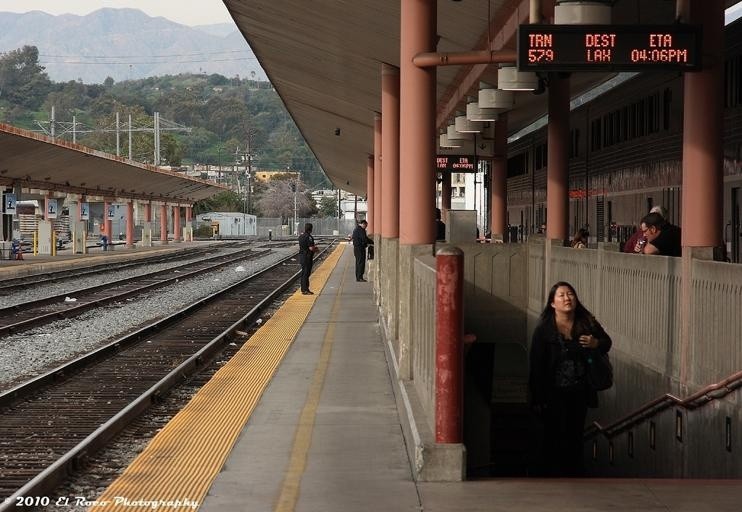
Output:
<box><xmin>581</xmin><ymin>309</ymin><xmax>614</xmax><ymax>391</ymax></box>
<box><xmin>366</xmin><ymin>243</ymin><xmax>374</xmax><ymax>262</ymax></box>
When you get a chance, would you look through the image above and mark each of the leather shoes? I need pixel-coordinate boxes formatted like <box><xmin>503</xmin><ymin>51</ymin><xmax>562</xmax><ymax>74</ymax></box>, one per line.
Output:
<box><xmin>356</xmin><ymin>278</ymin><xmax>367</xmax><ymax>282</ymax></box>
<box><xmin>301</xmin><ymin>289</ymin><xmax>314</xmax><ymax>295</ymax></box>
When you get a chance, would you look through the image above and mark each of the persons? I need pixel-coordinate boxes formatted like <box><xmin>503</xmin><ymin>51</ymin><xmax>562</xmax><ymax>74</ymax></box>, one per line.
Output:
<box><xmin>504</xmin><ymin>224</ymin><xmax>523</xmax><ymax>244</ymax></box>
<box><xmin>537</xmin><ymin>224</ymin><xmax>546</xmax><ymax>233</ymax></box>
<box><xmin>352</xmin><ymin>220</ymin><xmax>374</xmax><ymax>282</ymax></box>
<box><xmin>436</xmin><ymin>208</ymin><xmax>446</xmax><ymax>240</ymax></box>
<box><xmin>528</xmin><ymin>282</ymin><xmax>613</xmax><ymax>477</ymax></box>
<box><xmin>571</xmin><ymin>229</ymin><xmax>590</xmax><ymax>249</ymax></box>
<box><xmin>464</xmin><ymin>316</ymin><xmax>495</xmax><ymax>478</ymax></box>
<box><xmin>299</xmin><ymin>224</ymin><xmax>319</xmax><ymax>294</ymax></box>
<box><xmin>101</xmin><ymin>234</ymin><xmax>107</xmax><ymax>250</ymax></box>
<box><xmin>623</xmin><ymin>205</ymin><xmax>669</xmax><ymax>252</ymax></box>
<box><xmin>641</xmin><ymin>212</ymin><xmax>681</xmax><ymax>256</ymax></box>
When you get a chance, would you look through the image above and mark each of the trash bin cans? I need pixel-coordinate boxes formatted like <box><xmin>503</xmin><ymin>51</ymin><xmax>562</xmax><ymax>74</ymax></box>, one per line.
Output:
<box><xmin>101</xmin><ymin>236</ymin><xmax>107</xmax><ymax>251</ymax></box>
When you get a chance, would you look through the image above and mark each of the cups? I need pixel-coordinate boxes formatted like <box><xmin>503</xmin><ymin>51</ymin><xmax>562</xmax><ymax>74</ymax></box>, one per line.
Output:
<box><xmin>637</xmin><ymin>237</ymin><xmax>648</xmax><ymax>253</ymax></box>
